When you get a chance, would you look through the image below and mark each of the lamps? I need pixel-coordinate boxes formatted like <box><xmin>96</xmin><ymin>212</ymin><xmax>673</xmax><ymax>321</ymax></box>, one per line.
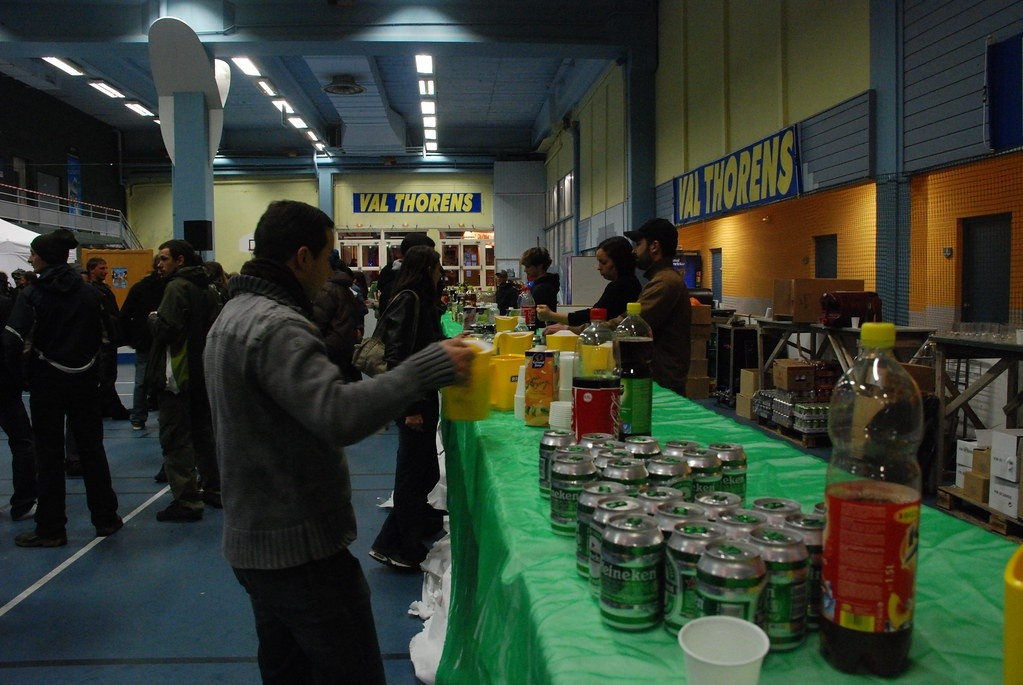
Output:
<box><xmin>41</xmin><ymin>54</ymin><xmax>443</xmax><ymax>156</ymax></box>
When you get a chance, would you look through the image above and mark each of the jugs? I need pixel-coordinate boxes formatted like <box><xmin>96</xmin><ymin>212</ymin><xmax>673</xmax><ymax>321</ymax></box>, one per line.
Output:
<box><xmin>442</xmin><ymin>316</ymin><xmax>535</xmax><ymax>420</ymax></box>
<box><xmin>546</xmin><ymin>329</ymin><xmax>616</xmax><ymax>376</ymax></box>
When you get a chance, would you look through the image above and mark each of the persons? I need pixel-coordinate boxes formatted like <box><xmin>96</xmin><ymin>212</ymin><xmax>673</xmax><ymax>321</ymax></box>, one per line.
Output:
<box><xmin>542</xmin><ymin>217</ymin><xmax>691</xmax><ymax>399</ymax></box>
<box><xmin>0</xmin><ymin>227</ymin><xmax>124</xmax><ymax>547</ymax></box>
<box><xmin>368</xmin><ymin>232</ymin><xmax>469</xmax><ymax>570</ymax></box>
<box><xmin>535</xmin><ymin>235</ymin><xmax>643</xmax><ymax>328</ymax></box>
<box><xmin>495</xmin><ymin>271</ymin><xmax>518</xmax><ymax>317</ymax></box>
<box><xmin>519</xmin><ymin>246</ymin><xmax>561</xmax><ymax>334</ymax></box>
<box><xmin>148</xmin><ymin>239</ymin><xmax>223</xmax><ymax>521</ymax></box>
<box><xmin>200</xmin><ymin>199</ymin><xmax>475</xmax><ymax>685</ymax></box>
<box><xmin>0</xmin><ymin>239</ymin><xmax>368</xmax><ymax>519</ymax></box>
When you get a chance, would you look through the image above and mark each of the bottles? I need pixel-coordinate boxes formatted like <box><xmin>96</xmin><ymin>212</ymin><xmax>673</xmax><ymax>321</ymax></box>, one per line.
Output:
<box><xmin>448</xmin><ymin>285</ymin><xmax>500</xmax><ymax>343</ymax></box>
<box><xmin>511</xmin><ymin>281</ymin><xmax>548</xmax><ymax>346</ymax></box>
<box><xmin>570</xmin><ymin>308</ymin><xmax>621</xmax><ymax>444</ymax></box>
<box><xmin>614</xmin><ymin>302</ymin><xmax>654</xmax><ymax>442</ymax></box>
<box><xmin>818</xmin><ymin>322</ymin><xmax>922</xmax><ymax>679</ymax></box>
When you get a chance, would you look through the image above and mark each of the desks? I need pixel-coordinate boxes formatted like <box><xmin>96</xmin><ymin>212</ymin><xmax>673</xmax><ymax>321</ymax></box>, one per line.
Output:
<box><xmin>432</xmin><ymin>310</ymin><xmax>1023</xmax><ymax>685</ymax></box>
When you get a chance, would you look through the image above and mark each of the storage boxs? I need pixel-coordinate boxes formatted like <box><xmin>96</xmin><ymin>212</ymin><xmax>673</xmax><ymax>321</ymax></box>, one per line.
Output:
<box><xmin>689</xmin><ymin>278</ymin><xmax>863</xmax><ymax>433</ymax></box>
<box><xmin>956</xmin><ymin>427</ymin><xmax>1023</xmax><ymax>520</ymax></box>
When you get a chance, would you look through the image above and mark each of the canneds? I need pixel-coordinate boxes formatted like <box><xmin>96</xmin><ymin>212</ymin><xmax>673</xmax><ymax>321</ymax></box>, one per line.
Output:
<box><xmin>755</xmin><ymin>390</ymin><xmax>849</xmax><ymax>429</ymax></box>
<box><xmin>537</xmin><ymin>429</ymin><xmax>747</xmax><ymax>538</ymax></box>
<box><xmin>575</xmin><ymin>481</ymin><xmax>828</xmax><ymax>653</ymax></box>
<box><xmin>471</xmin><ymin>324</ymin><xmax>541</xmax><ymax>346</ymax></box>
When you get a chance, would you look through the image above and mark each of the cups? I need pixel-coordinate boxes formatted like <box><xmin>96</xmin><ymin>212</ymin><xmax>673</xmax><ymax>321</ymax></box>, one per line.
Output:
<box><xmin>513</xmin><ymin>351</ymin><xmax>574</xmax><ymax>432</ymax></box>
<box><xmin>936</xmin><ymin>318</ymin><xmax>1018</xmax><ymax>342</ymax></box>
<box><xmin>1004</xmin><ymin>545</ymin><xmax>1023</xmax><ymax>684</ymax></box>
<box><xmin>677</xmin><ymin>615</ymin><xmax>770</xmax><ymax>685</ymax></box>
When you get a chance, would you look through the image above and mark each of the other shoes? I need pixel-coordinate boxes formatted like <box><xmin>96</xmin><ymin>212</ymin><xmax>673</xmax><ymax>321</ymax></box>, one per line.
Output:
<box><xmin>112</xmin><ymin>409</ymin><xmax>132</xmax><ymax>420</ymax></box>
<box><xmin>13</xmin><ymin>503</ymin><xmax>38</xmax><ymax>520</ymax></box>
<box><xmin>15</xmin><ymin>531</ymin><xmax>67</xmax><ymax>547</ymax></box>
<box><xmin>156</xmin><ymin>498</ymin><xmax>205</xmax><ymax>522</ymax></box>
<box><xmin>65</xmin><ymin>470</ymin><xmax>84</xmax><ymax>479</ymax></box>
<box><xmin>201</xmin><ymin>490</ymin><xmax>222</xmax><ymax>509</ymax></box>
<box><xmin>149</xmin><ymin>399</ymin><xmax>158</xmax><ymax>411</ymax></box>
<box><xmin>134</xmin><ymin>424</ymin><xmax>146</xmax><ymax>430</ymax></box>
<box><xmin>155</xmin><ymin>463</ymin><xmax>172</xmax><ymax>483</ymax></box>
<box><xmin>96</xmin><ymin>515</ymin><xmax>123</xmax><ymax>537</ymax></box>
<box><xmin>368</xmin><ymin>547</ymin><xmax>412</xmax><ymax>570</ymax></box>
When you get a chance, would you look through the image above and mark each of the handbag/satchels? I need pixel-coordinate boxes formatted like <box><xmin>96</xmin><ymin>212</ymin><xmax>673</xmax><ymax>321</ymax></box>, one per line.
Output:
<box><xmin>351</xmin><ymin>289</ymin><xmax>420</xmax><ymax>378</ymax></box>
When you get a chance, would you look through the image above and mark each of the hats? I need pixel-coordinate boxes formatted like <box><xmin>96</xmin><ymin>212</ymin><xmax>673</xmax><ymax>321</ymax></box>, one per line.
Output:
<box><xmin>31</xmin><ymin>229</ymin><xmax>80</xmax><ymax>261</ymax></box>
<box><xmin>623</xmin><ymin>219</ymin><xmax>678</xmax><ymax>242</ymax></box>
<box><xmin>11</xmin><ymin>269</ymin><xmax>26</xmax><ymax>279</ymax></box>
<box><xmin>496</xmin><ymin>270</ymin><xmax>509</xmax><ymax>278</ymax></box>
